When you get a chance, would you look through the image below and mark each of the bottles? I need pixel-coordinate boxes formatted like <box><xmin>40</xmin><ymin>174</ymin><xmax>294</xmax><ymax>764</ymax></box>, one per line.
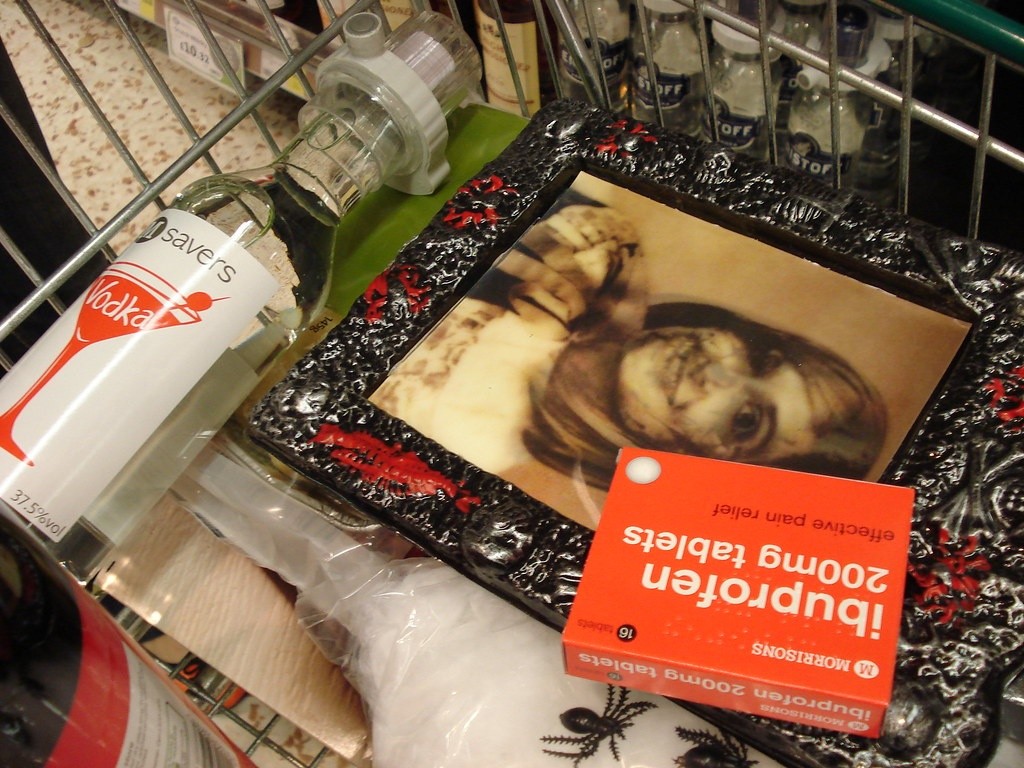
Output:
<box><xmin>0</xmin><ymin>0</ymin><xmax>934</xmax><ymax>768</ymax></box>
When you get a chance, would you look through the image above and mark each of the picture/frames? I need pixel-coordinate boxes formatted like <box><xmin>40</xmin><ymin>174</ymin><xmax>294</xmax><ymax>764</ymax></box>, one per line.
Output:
<box><xmin>249</xmin><ymin>94</ymin><xmax>1024</xmax><ymax>768</ymax></box>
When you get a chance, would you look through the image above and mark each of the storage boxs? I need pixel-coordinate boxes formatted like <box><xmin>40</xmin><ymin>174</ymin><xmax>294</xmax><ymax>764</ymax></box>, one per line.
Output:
<box><xmin>559</xmin><ymin>443</ymin><xmax>915</xmax><ymax>739</ymax></box>
